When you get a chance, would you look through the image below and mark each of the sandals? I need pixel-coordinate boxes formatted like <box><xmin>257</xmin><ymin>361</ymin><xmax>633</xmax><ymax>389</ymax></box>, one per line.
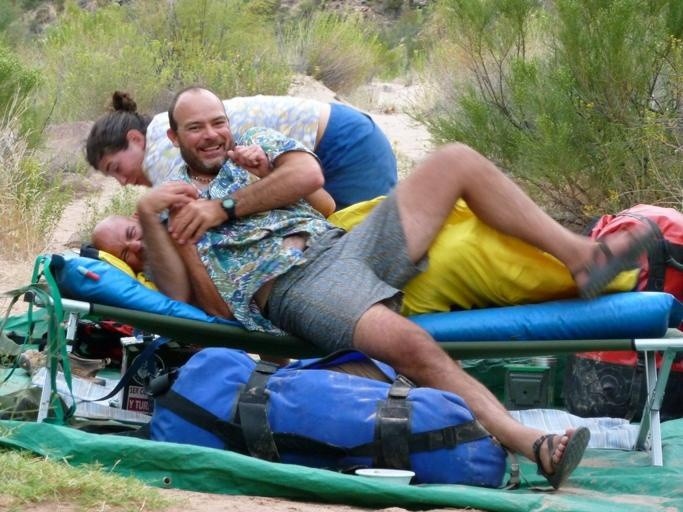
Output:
<box><xmin>570</xmin><ymin>226</ymin><xmax>655</xmax><ymax>298</ymax></box>
<box><xmin>532</xmin><ymin>427</ymin><xmax>589</xmax><ymax>492</ymax></box>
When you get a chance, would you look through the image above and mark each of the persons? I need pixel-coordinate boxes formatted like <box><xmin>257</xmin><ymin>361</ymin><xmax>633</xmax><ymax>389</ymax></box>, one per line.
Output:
<box><xmin>138</xmin><ymin>86</ymin><xmax>656</xmax><ymax>490</ymax></box>
<box><xmin>86</xmin><ymin>90</ymin><xmax>398</xmax><ymax>212</ymax></box>
<box><xmin>91</xmin><ymin>142</ymin><xmax>642</xmax><ymax>320</ymax></box>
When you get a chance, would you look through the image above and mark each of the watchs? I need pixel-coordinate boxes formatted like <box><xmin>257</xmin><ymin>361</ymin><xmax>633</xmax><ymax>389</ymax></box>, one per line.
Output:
<box><xmin>221</xmin><ymin>194</ymin><xmax>238</xmax><ymax>221</ymax></box>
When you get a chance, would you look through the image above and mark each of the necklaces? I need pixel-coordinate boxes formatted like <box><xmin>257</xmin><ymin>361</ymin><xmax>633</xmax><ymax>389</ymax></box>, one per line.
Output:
<box><xmin>189</xmin><ymin>174</ymin><xmax>215</xmax><ymax>185</ymax></box>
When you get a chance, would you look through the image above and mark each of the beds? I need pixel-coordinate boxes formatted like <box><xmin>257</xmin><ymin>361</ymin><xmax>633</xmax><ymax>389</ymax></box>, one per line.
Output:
<box><xmin>26</xmin><ymin>256</ymin><xmax>682</xmax><ymax>469</ymax></box>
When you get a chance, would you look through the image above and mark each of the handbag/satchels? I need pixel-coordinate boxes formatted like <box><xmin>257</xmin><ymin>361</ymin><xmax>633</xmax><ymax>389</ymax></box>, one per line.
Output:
<box><xmin>150</xmin><ymin>345</ymin><xmax>506</xmax><ymax>487</ymax></box>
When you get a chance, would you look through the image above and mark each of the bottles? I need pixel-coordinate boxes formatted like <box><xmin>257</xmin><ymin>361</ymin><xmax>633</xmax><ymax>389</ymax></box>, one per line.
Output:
<box><xmin>68</xmin><ymin>353</ymin><xmax>106</xmax><ymax>376</ymax></box>
